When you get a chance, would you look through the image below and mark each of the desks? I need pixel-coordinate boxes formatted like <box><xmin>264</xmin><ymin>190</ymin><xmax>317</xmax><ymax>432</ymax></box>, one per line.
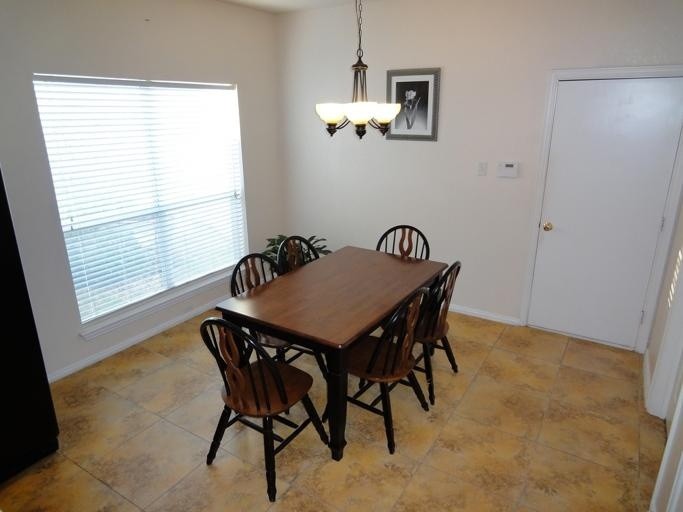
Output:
<box><xmin>214</xmin><ymin>245</ymin><xmax>449</xmax><ymax>462</ymax></box>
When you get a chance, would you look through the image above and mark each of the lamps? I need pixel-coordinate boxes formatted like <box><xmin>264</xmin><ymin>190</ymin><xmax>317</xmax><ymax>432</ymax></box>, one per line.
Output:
<box><xmin>316</xmin><ymin>0</ymin><xmax>402</xmax><ymax>139</ymax></box>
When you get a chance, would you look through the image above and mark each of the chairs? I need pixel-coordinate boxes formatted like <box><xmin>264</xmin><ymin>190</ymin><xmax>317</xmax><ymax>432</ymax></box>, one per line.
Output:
<box><xmin>230</xmin><ymin>254</ymin><xmax>331</xmax><ymax>384</ymax></box>
<box><xmin>381</xmin><ymin>261</ymin><xmax>463</xmax><ymax>405</ymax></box>
<box><xmin>199</xmin><ymin>316</ymin><xmax>330</xmax><ymax>502</ymax></box>
<box><xmin>322</xmin><ymin>287</ymin><xmax>429</xmax><ymax>455</ymax></box>
<box><xmin>376</xmin><ymin>225</ymin><xmax>429</xmax><ymax>262</ymax></box>
<box><xmin>276</xmin><ymin>236</ymin><xmax>319</xmax><ymax>275</ymax></box>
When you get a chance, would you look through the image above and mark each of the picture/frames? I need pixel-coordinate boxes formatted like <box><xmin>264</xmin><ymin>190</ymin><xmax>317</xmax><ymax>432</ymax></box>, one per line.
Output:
<box><xmin>385</xmin><ymin>67</ymin><xmax>441</xmax><ymax>141</ymax></box>
